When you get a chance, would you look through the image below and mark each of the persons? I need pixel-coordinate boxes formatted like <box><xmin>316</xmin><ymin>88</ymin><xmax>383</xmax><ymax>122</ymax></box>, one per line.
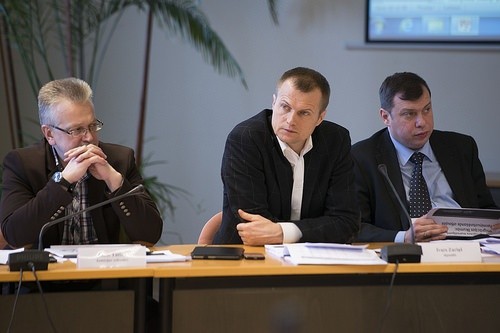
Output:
<box><xmin>0</xmin><ymin>78</ymin><xmax>163</xmax><ymax>248</ymax></box>
<box><xmin>212</xmin><ymin>67</ymin><xmax>360</xmax><ymax>245</ymax></box>
<box><xmin>351</xmin><ymin>71</ymin><xmax>500</xmax><ymax>243</ymax></box>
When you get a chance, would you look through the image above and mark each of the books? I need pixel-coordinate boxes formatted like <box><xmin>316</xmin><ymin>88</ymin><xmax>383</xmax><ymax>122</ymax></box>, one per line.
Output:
<box><xmin>192</xmin><ymin>247</ymin><xmax>244</xmax><ymax>260</ymax></box>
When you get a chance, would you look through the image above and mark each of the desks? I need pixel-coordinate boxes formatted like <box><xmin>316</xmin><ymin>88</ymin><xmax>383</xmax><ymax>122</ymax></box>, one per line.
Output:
<box><xmin>0</xmin><ymin>242</ymin><xmax>500</xmax><ymax>333</ymax></box>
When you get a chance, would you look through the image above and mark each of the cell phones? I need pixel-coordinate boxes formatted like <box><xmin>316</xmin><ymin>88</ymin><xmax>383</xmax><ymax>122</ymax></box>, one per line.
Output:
<box><xmin>244</xmin><ymin>252</ymin><xmax>265</xmax><ymax>260</ymax></box>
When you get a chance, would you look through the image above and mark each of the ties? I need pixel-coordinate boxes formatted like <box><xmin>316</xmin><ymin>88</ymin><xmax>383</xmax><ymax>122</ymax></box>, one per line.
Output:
<box><xmin>410</xmin><ymin>153</ymin><xmax>431</xmax><ymax>219</ymax></box>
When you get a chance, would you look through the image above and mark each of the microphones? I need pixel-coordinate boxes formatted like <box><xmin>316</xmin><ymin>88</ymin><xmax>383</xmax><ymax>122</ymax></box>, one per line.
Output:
<box><xmin>38</xmin><ymin>183</ymin><xmax>145</xmax><ymax>251</ymax></box>
<box><xmin>378</xmin><ymin>163</ymin><xmax>415</xmax><ymax>245</ymax></box>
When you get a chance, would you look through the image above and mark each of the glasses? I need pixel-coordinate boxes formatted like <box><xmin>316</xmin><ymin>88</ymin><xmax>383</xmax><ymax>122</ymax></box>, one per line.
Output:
<box><xmin>49</xmin><ymin>118</ymin><xmax>104</xmax><ymax>137</ymax></box>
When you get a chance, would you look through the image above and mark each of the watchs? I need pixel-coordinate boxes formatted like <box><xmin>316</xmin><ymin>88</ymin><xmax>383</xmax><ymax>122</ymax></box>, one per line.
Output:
<box><xmin>54</xmin><ymin>172</ymin><xmax>71</xmax><ymax>188</ymax></box>
